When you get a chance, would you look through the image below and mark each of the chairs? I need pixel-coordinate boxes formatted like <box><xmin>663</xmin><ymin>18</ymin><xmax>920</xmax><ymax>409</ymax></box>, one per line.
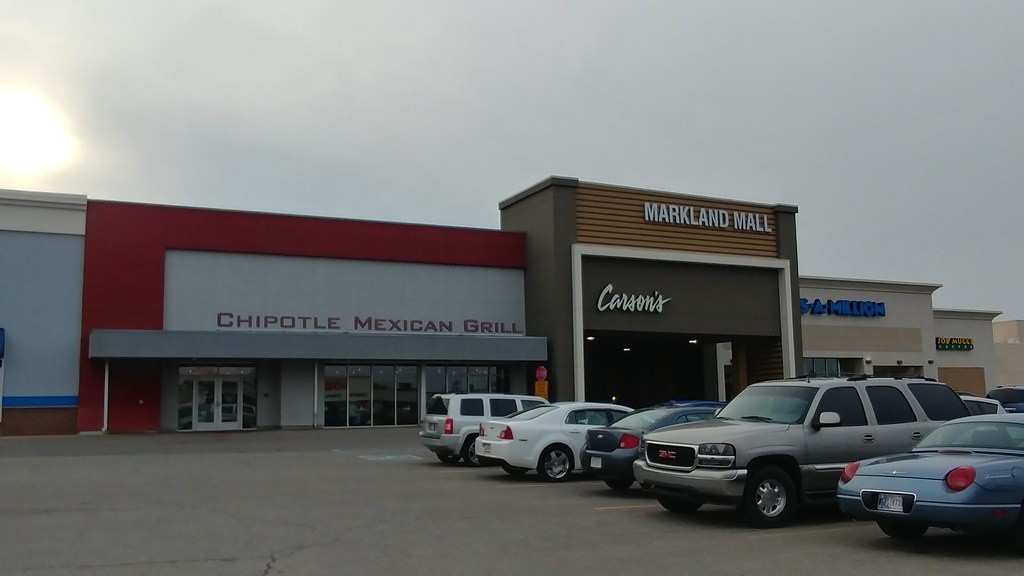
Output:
<box><xmin>586</xmin><ymin>415</ymin><xmax>601</xmax><ymax>427</ymax></box>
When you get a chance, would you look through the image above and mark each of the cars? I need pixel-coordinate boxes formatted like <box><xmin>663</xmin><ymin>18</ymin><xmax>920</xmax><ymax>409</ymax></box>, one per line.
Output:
<box><xmin>580</xmin><ymin>404</ymin><xmax>729</xmax><ymax>495</ymax></box>
<box><xmin>836</xmin><ymin>414</ymin><xmax>1024</xmax><ymax>544</ymax></box>
<box><xmin>475</xmin><ymin>401</ymin><xmax>640</xmax><ymax>481</ymax></box>
<box><xmin>663</xmin><ymin>400</ymin><xmax>727</xmax><ymax>411</ymax></box>
<box><xmin>177</xmin><ymin>395</ymin><xmax>418</xmax><ymax>431</ymax></box>
<box><xmin>956</xmin><ymin>383</ymin><xmax>1023</xmax><ymax>421</ymax></box>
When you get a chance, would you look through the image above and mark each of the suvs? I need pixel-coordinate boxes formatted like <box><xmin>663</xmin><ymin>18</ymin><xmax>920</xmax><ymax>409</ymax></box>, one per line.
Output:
<box><xmin>419</xmin><ymin>392</ymin><xmax>551</xmax><ymax>464</ymax></box>
<box><xmin>632</xmin><ymin>376</ymin><xmax>974</xmax><ymax>530</ymax></box>
